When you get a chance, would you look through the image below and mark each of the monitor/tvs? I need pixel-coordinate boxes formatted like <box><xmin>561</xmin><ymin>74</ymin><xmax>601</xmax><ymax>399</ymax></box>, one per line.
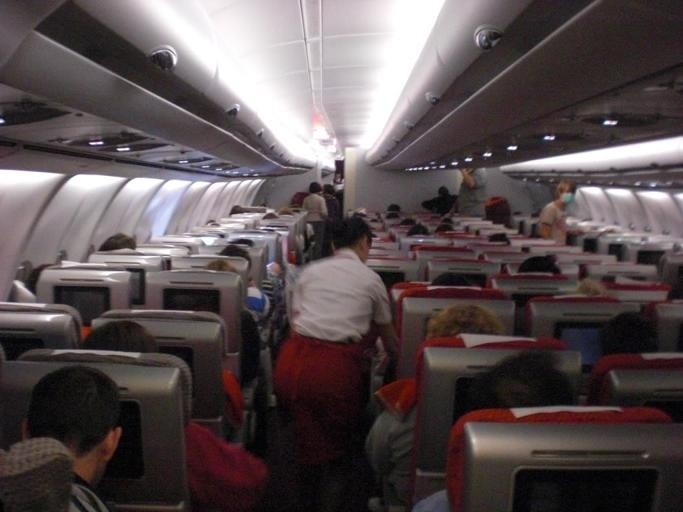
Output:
<box><xmin>512</xmin><ymin>294</ymin><xmax>546</xmax><ymax>307</ymax></box>
<box><xmin>555</xmin><ymin>319</ymin><xmax>615</xmax><ymax>372</ymax></box>
<box><xmin>511</xmin><ymin>466</ymin><xmax>659</xmax><ymax>512</ymax></box>
<box><xmin>452</xmin><ymin>376</ymin><xmax>500</xmax><ymax>426</ymax></box>
<box><xmin>163</xmin><ymin>286</ymin><xmax>220</xmax><ymax>314</ymax></box>
<box><xmin>464</xmin><ymin>273</ymin><xmax>487</xmax><ymax>286</ymax></box>
<box><xmin>160</xmin><ymin>344</ymin><xmax>196</xmax><ymax>375</ymax></box>
<box><xmin>125</xmin><ymin>271</ymin><xmax>144</xmax><ymax>304</ymax></box>
<box><xmin>642</xmin><ymin>399</ymin><xmax>683</xmax><ymax>424</ymax></box>
<box><xmin>55</xmin><ymin>284</ymin><xmax>111</xmax><ymax>326</ymax></box>
<box><xmin>99</xmin><ymin>400</ymin><xmax>143</xmax><ymax>484</ymax></box>
<box><xmin>3</xmin><ymin>337</ymin><xmax>42</xmax><ymax>357</ymax></box>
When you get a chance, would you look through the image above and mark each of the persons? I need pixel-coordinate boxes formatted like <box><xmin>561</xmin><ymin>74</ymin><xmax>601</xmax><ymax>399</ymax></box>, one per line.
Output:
<box><xmin>0</xmin><ymin>166</ymin><xmax>683</xmax><ymax>512</ymax></box>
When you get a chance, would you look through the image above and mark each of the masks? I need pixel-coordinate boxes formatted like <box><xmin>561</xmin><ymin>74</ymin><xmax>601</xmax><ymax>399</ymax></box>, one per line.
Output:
<box><xmin>560</xmin><ymin>192</ymin><xmax>573</xmax><ymax>204</ymax></box>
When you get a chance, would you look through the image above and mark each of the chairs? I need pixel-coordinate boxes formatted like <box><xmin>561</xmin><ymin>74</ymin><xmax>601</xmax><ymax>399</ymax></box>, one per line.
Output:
<box><xmin>351</xmin><ymin>204</ymin><xmax>682</xmax><ymax>512</ymax></box>
<box><xmin>0</xmin><ymin>204</ymin><xmax>317</xmax><ymax>506</ymax></box>
